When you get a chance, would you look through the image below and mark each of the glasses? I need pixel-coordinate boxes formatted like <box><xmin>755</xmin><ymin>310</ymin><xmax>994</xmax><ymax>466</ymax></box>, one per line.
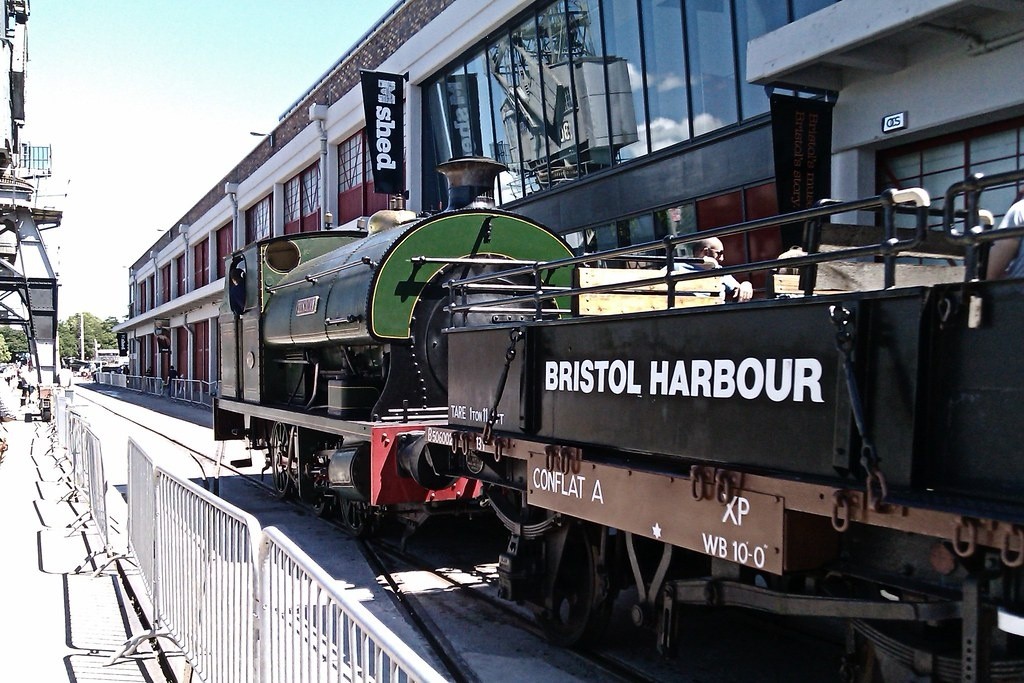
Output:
<box><xmin>708</xmin><ymin>248</ymin><xmax>723</xmax><ymax>255</ymax></box>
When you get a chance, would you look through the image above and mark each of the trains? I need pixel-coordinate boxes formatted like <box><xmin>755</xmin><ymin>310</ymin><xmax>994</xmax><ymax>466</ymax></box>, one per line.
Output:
<box><xmin>209</xmin><ymin>156</ymin><xmax>1024</xmax><ymax>683</ymax></box>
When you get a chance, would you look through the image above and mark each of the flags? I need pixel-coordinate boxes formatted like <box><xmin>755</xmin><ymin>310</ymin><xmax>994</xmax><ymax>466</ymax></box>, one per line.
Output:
<box><xmin>436</xmin><ymin>73</ymin><xmax>486</xmax><ymax>159</ymax></box>
<box><xmin>359</xmin><ymin>69</ymin><xmax>405</xmax><ymax>198</ymax></box>
<box><xmin>116</xmin><ymin>333</ymin><xmax>129</xmax><ymax>357</ymax></box>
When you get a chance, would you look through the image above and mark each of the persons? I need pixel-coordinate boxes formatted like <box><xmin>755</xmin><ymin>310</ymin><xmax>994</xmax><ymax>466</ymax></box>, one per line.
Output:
<box><xmin>178</xmin><ymin>373</ymin><xmax>186</xmax><ymax>395</ymax></box>
<box><xmin>986</xmin><ymin>198</ymin><xmax>1024</xmax><ymax>280</ymax></box>
<box><xmin>160</xmin><ymin>378</ymin><xmax>168</xmax><ymax>396</ymax></box>
<box><xmin>92</xmin><ymin>367</ymin><xmax>98</xmax><ymax>383</ymax></box>
<box><xmin>146</xmin><ymin>367</ymin><xmax>153</xmax><ymax>386</ymax></box>
<box><xmin>16</xmin><ymin>359</ymin><xmax>36</xmax><ymax>408</ymax></box>
<box><xmin>123</xmin><ymin>364</ymin><xmax>130</xmax><ymax>384</ymax></box>
<box><xmin>117</xmin><ymin>365</ymin><xmax>123</xmax><ymax>374</ymax></box>
<box><xmin>167</xmin><ymin>366</ymin><xmax>179</xmax><ymax>397</ymax></box>
<box><xmin>660</xmin><ymin>235</ymin><xmax>753</xmax><ymax>303</ymax></box>
